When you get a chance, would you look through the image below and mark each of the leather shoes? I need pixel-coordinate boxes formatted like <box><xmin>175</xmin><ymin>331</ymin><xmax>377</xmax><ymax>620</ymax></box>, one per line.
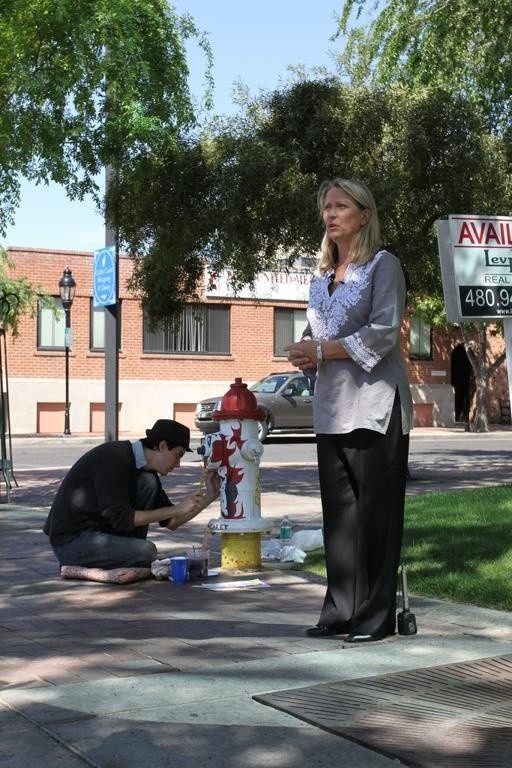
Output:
<box><xmin>305</xmin><ymin>625</ymin><xmax>332</xmax><ymax>637</ymax></box>
<box><xmin>343</xmin><ymin>632</ymin><xmax>377</xmax><ymax>643</ymax></box>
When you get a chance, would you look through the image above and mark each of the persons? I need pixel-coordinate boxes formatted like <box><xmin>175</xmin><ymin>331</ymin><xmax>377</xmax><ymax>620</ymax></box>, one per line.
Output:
<box><xmin>448</xmin><ymin>342</ymin><xmax>475</xmax><ymax>422</ymax></box>
<box><xmin>282</xmin><ymin>179</ymin><xmax>414</xmax><ymax>644</ymax></box>
<box><xmin>42</xmin><ymin>418</ymin><xmax>221</xmax><ymax>585</ymax></box>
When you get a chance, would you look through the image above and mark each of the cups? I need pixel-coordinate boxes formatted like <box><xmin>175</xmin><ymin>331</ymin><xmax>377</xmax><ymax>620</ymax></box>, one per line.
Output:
<box><xmin>167</xmin><ymin>557</ymin><xmax>209</xmax><ymax>583</ymax></box>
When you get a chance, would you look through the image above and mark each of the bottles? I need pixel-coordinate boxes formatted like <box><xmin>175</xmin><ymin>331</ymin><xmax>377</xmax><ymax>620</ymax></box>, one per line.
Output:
<box><xmin>280</xmin><ymin>515</ymin><xmax>294</xmax><ymax>562</ymax></box>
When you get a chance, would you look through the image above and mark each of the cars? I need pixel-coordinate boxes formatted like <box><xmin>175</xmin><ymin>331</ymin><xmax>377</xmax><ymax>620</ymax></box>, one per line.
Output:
<box><xmin>195</xmin><ymin>371</ymin><xmax>313</xmax><ymax>444</ymax></box>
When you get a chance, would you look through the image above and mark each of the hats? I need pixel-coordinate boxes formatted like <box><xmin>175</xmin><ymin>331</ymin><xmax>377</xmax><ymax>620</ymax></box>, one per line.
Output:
<box><xmin>145</xmin><ymin>418</ymin><xmax>194</xmax><ymax>453</ymax></box>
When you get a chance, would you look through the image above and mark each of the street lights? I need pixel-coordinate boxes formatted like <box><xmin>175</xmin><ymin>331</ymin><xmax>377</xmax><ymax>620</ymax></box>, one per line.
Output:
<box><xmin>58</xmin><ymin>268</ymin><xmax>76</xmax><ymax>435</ymax></box>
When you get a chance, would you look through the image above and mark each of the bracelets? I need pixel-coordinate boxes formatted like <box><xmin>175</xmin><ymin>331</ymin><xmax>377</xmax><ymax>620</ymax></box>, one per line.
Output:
<box><xmin>316</xmin><ymin>343</ymin><xmax>327</xmax><ymax>366</ymax></box>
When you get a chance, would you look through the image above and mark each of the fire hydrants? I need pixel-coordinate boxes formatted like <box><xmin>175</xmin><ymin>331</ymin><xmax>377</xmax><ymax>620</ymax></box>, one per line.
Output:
<box><xmin>197</xmin><ymin>377</ymin><xmax>275</xmax><ymax>575</ymax></box>
